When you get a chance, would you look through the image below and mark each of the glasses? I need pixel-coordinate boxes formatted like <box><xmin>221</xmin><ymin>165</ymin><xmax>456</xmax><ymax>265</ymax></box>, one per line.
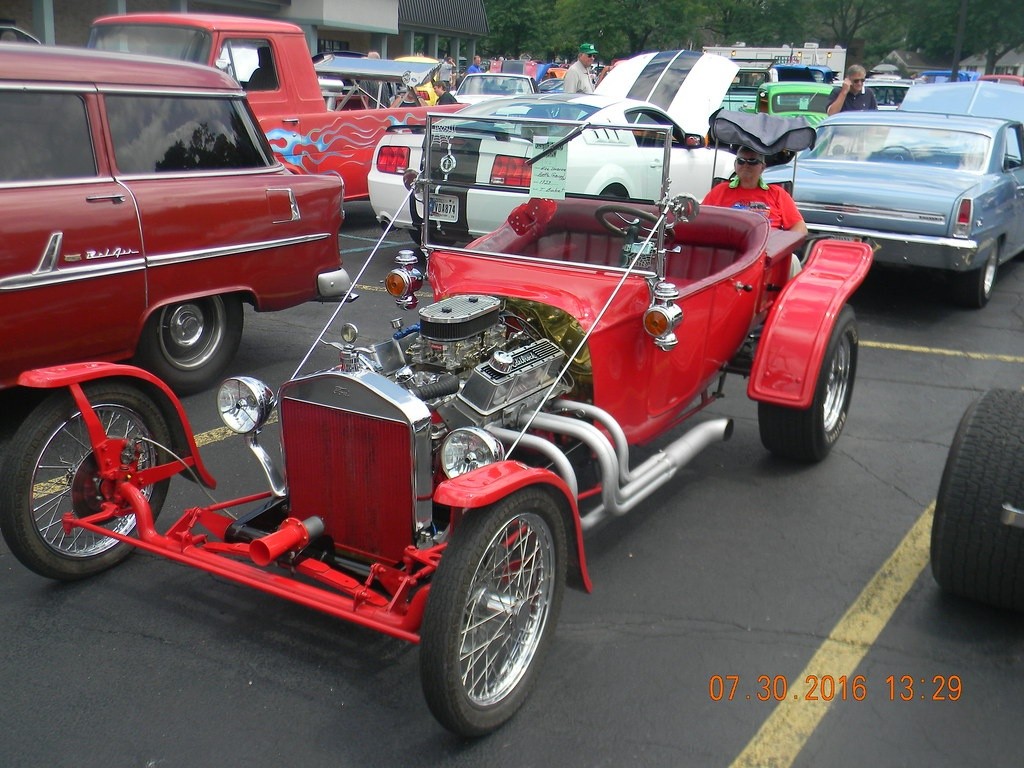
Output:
<box><xmin>736</xmin><ymin>157</ymin><xmax>764</xmax><ymax>166</ymax></box>
<box><xmin>850</xmin><ymin>79</ymin><xmax>865</xmax><ymax>84</ymax></box>
<box><xmin>585</xmin><ymin>53</ymin><xmax>596</xmax><ymax>59</ymax></box>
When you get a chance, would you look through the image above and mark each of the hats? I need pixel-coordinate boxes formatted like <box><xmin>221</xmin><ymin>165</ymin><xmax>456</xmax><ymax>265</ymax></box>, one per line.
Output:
<box><xmin>579</xmin><ymin>43</ymin><xmax>599</xmax><ymax>54</ymax></box>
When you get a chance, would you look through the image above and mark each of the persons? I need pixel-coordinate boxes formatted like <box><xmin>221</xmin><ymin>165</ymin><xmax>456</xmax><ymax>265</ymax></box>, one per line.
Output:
<box><xmin>417</xmin><ymin>51</ymin><xmax>424</xmax><ymax>57</ymax></box>
<box><xmin>825</xmin><ymin>65</ymin><xmax>879</xmax><ymax>155</ymax></box>
<box><xmin>431</xmin><ymin>79</ymin><xmax>457</xmax><ymax>105</ymax></box>
<box><xmin>563</xmin><ymin>43</ymin><xmax>626</xmax><ymax>94</ymax></box>
<box><xmin>448</xmin><ymin>55</ymin><xmax>543</xmax><ymax>94</ymax></box>
<box><xmin>893</xmin><ymin>71</ymin><xmax>928</xmax><ymax>105</ymax></box>
<box><xmin>358</xmin><ymin>52</ymin><xmax>390</xmax><ymax>108</ymax></box>
<box><xmin>389</xmin><ymin>86</ymin><xmax>428</xmax><ymax>108</ymax></box>
<box><xmin>701</xmin><ymin>145</ymin><xmax>808</xmax><ymax>278</ymax></box>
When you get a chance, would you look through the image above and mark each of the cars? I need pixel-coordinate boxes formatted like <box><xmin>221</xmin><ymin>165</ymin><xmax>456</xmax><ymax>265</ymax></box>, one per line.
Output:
<box><xmin>1</xmin><ymin>42</ymin><xmax>344</xmax><ymax>396</ymax></box>
<box><xmin>755</xmin><ymin>80</ymin><xmax>1024</xmax><ymax>310</ymax></box>
<box><xmin>367</xmin><ymin>47</ymin><xmax>737</xmax><ymax>249</ymax></box>
<box><xmin>373</xmin><ymin>51</ymin><xmax>1023</xmax><ymax>131</ymax></box>
<box><xmin>0</xmin><ymin>111</ymin><xmax>875</xmax><ymax>739</ymax></box>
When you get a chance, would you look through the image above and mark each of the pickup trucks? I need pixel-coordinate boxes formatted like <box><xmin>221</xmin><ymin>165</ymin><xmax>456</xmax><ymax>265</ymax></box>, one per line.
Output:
<box><xmin>87</xmin><ymin>13</ymin><xmax>468</xmax><ymax>206</ymax></box>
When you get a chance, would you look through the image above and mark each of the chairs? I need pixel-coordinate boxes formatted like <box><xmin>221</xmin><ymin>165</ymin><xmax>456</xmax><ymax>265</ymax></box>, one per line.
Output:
<box><xmin>246</xmin><ymin>46</ymin><xmax>279</xmax><ymax>91</ymax></box>
